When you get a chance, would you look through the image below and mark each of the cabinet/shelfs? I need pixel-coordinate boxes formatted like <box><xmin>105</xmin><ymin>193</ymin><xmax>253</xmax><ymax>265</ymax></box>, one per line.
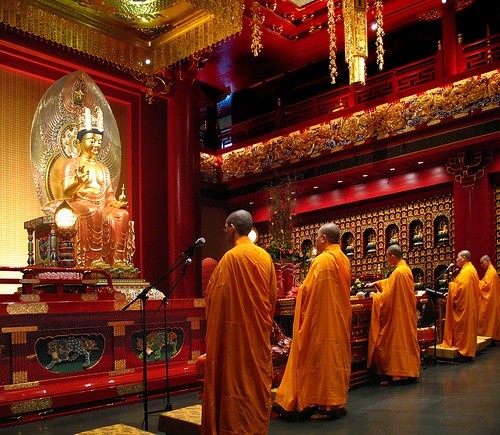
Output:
<box><xmin>255</xmin><ymin>292</ymin><xmax>430</xmax><ymax>407</ymax></box>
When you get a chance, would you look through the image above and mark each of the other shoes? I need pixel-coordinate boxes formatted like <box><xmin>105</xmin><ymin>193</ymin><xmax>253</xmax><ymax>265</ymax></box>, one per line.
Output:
<box><xmin>380</xmin><ymin>378</ymin><xmax>408</xmax><ymax>386</ymax></box>
<box><xmin>310</xmin><ymin>407</ymin><xmax>346</xmax><ymax>421</ymax></box>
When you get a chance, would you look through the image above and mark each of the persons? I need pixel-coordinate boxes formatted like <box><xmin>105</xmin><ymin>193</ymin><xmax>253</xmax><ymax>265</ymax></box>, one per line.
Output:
<box><xmin>275</xmin><ymin>223</ymin><xmax>353</xmax><ymax>418</ymax></box>
<box><xmin>60</xmin><ymin>107</ymin><xmax>137</xmax><ymax>272</ymax></box>
<box><xmin>203</xmin><ymin>211</ymin><xmax>277</xmax><ymax>435</ymax></box>
<box><xmin>474</xmin><ymin>255</ymin><xmax>500</xmax><ymax>341</ymax></box>
<box><xmin>445</xmin><ymin>250</ymin><xmax>482</xmax><ymax>359</ymax></box>
<box><xmin>363</xmin><ymin>245</ymin><xmax>422</xmax><ymax>386</ymax></box>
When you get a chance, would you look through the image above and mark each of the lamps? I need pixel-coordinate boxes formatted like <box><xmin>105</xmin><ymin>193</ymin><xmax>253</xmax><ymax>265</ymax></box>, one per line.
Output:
<box><xmin>53</xmin><ymin>201</ymin><xmax>80</xmax><ymax>243</ymax></box>
<box><xmin>327</xmin><ymin>0</ymin><xmax>385</xmax><ymax>87</ymax></box>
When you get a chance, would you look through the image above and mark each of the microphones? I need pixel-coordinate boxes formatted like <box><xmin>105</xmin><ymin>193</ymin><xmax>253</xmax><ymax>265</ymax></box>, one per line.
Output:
<box><xmin>180</xmin><ymin>238</ymin><xmax>206</xmax><ymax>257</ymax></box>
<box><xmin>445</xmin><ymin>263</ymin><xmax>454</xmax><ymax>272</ymax></box>
<box><xmin>180</xmin><ymin>259</ymin><xmax>192</xmax><ymax>274</ymax></box>
